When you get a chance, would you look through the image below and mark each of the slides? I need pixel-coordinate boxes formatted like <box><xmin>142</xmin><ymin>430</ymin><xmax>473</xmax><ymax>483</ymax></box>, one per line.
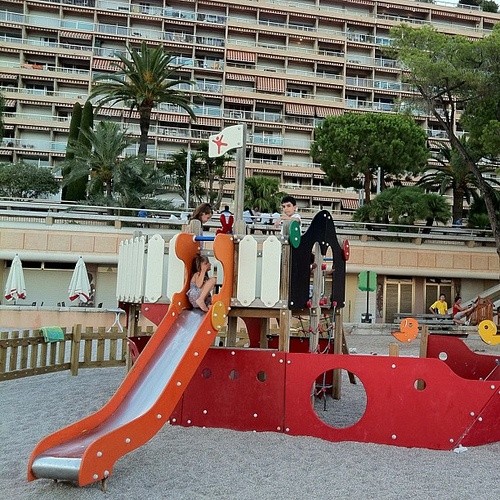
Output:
<box><xmin>27</xmin><ymin>233</ymin><xmax>234</xmax><ymax>488</ymax></box>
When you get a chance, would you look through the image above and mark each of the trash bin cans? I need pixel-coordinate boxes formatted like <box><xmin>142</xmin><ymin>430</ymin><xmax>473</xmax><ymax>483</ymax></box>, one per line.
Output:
<box><xmin>362</xmin><ymin>313</ymin><xmax>373</xmax><ymax>323</ymax></box>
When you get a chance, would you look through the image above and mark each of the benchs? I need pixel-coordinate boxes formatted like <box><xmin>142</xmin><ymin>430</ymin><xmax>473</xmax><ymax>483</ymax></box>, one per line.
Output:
<box><xmin>392</xmin><ymin>314</ymin><xmax>454</xmax><ymax>328</ymax></box>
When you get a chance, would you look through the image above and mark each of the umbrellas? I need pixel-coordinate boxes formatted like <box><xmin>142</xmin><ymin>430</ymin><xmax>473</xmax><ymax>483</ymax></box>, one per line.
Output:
<box><xmin>4</xmin><ymin>253</ymin><xmax>26</xmax><ymax>305</ymax></box>
<box><xmin>67</xmin><ymin>255</ymin><xmax>92</xmax><ymax>306</ymax></box>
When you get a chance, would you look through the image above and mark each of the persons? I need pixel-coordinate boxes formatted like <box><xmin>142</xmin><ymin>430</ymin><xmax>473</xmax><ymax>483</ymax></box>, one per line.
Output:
<box><xmin>186</xmin><ymin>252</ymin><xmax>215</xmax><ymax>312</ymax></box>
<box><xmin>453</xmin><ymin>296</ymin><xmax>477</xmax><ymax>320</ymax></box>
<box><xmin>138</xmin><ymin>206</ymin><xmax>147</xmax><ymax>228</ymax></box>
<box><xmin>429</xmin><ymin>294</ymin><xmax>448</xmax><ymax>315</ymax></box>
<box><xmin>220</xmin><ymin>206</ymin><xmax>232</xmax><ymax>216</ymax></box>
<box><xmin>262</xmin><ymin>209</ymin><xmax>270</xmax><ymax>235</ymax></box>
<box><xmin>275</xmin><ymin>196</ymin><xmax>301</xmax><ymax>236</ymax></box>
<box><xmin>269</xmin><ymin>209</ymin><xmax>281</xmax><ymax>235</ymax></box>
<box><xmin>243</xmin><ymin>208</ymin><xmax>254</xmax><ymax>234</ymax></box>
<box><xmin>183</xmin><ymin>203</ymin><xmax>213</xmax><ymax>248</ymax></box>
<box><xmin>250</xmin><ymin>207</ymin><xmax>261</xmax><ymax>234</ymax></box>
